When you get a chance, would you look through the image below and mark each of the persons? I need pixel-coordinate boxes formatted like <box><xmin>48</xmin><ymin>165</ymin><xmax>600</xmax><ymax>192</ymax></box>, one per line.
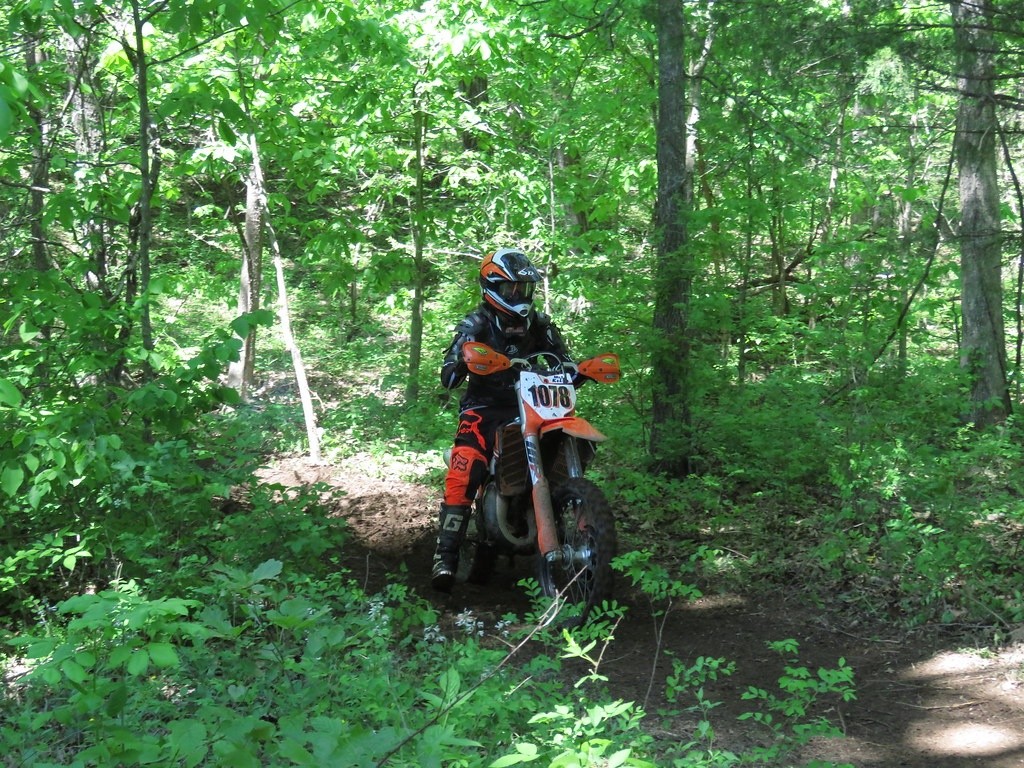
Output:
<box><xmin>430</xmin><ymin>247</ymin><xmax>623</xmax><ymax>590</ymax></box>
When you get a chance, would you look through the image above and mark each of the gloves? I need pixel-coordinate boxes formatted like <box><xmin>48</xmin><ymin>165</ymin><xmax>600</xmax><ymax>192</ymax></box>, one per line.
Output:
<box><xmin>455</xmin><ymin>358</ymin><xmax>471</xmax><ymax>376</ymax></box>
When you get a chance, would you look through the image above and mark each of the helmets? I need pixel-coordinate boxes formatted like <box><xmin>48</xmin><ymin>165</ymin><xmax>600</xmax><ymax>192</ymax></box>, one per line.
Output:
<box><xmin>480</xmin><ymin>249</ymin><xmax>539</xmax><ymax>321</ymax></box>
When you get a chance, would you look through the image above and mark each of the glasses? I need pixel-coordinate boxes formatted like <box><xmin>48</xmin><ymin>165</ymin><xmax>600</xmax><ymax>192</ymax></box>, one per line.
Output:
<box><xmin>480</xmin><ymin>272</ymin><xmax>536</xmax><ymax>302</ymax></box>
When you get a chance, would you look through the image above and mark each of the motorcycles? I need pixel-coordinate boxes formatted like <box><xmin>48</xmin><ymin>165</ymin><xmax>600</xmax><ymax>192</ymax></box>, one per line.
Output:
<box><xmin>443</xmin><ymin>341</ymin><xmax>621</xmax><ymax>636</ymax></box>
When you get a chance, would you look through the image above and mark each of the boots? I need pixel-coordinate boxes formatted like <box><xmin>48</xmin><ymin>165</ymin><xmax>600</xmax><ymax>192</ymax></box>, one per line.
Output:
<box><xmin>431</xmin><ymin>503</ymin><xmax>472</xmax><ymax>589</ymax></box>
